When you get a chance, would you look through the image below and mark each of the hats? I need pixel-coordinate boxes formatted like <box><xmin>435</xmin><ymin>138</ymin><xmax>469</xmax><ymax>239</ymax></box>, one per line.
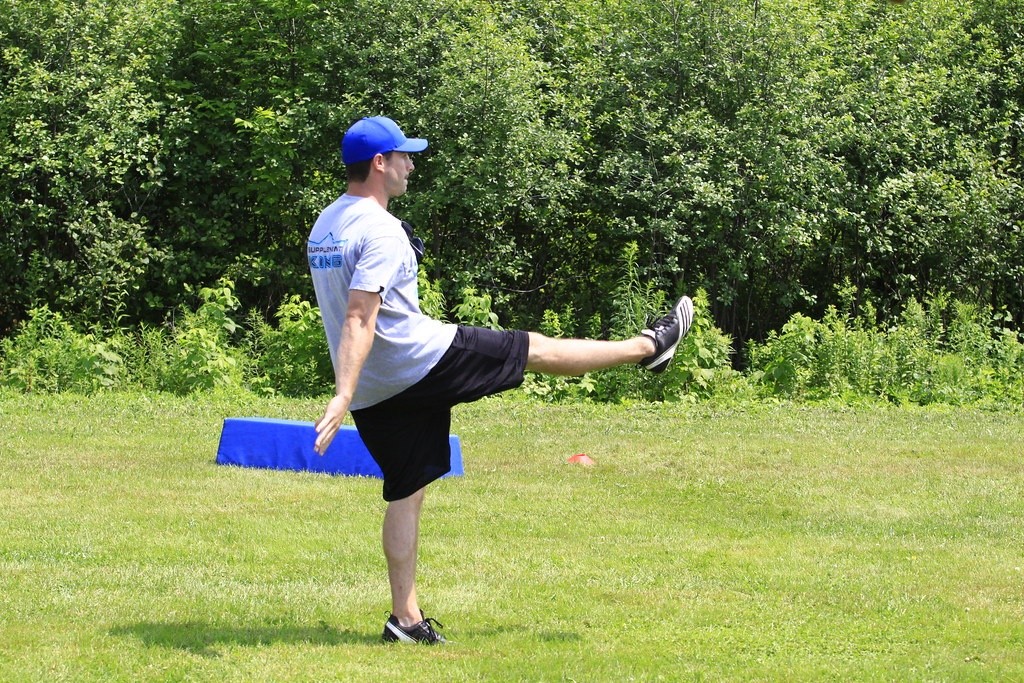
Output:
<box><xmin>342</xmin><ymin>116</ymin><xmax>428</xmax><ymax>164</ymax></box>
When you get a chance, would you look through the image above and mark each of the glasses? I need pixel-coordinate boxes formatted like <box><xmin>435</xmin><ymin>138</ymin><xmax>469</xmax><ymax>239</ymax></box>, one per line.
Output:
<box><xmin>401</xmin><ymin>220</ymin><xmax>425</xmax><ymax>263</ymax></box>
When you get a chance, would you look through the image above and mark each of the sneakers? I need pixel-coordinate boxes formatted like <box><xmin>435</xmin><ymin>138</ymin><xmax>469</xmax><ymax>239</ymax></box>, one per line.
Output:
<box><xmin>637</xmin><ymin>295</ymin><xmax>694</xmax><ymax>374</ymax></box>
<box><xmin>383</xmin><ymin>608</ymin><xmax>458</xmax><ymax>647</ymax></box>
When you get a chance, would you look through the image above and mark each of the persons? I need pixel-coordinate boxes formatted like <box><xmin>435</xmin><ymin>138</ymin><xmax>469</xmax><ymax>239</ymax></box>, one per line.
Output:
<box><xmin>306</xmin><ymin>116</ymin><xmax>696</xmax><ymax>649</ymax></box>
